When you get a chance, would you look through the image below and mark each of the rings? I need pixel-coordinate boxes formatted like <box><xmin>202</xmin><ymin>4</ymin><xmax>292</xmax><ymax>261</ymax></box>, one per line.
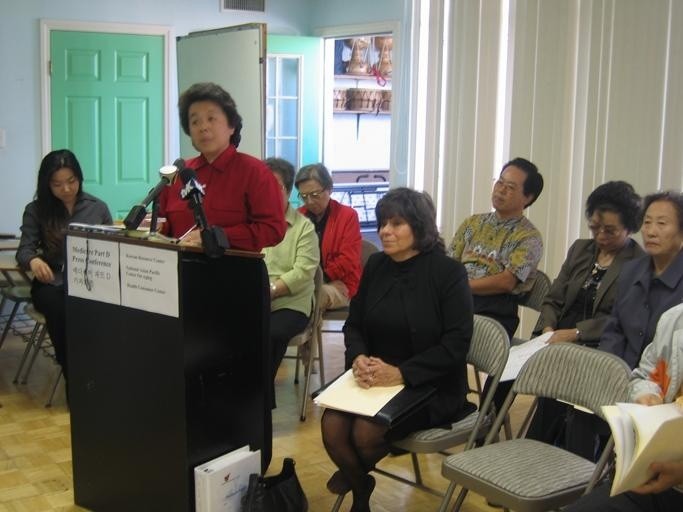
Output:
<box><xmin>189</xmin><ymin>236</ymin><xmax>194</xmax><ymax>241</ymax></box>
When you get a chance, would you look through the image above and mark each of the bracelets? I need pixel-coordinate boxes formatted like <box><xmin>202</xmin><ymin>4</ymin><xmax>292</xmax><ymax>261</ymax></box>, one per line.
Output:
<box><xmin>270</xmin><ymin>283</ymin><xmax>277</xmax><ymax>295</ymax></box>
<box><xmin>576</xmin><ymin>329</ymin><xmax>580</xmax><ymax>340</ymax></box>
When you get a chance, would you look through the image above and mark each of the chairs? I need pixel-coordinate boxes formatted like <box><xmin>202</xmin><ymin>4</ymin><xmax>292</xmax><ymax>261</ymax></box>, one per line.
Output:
<box><xmin>475</xmin><ymin>268</ymin><xmax>552</xmax><ymax>400</ymax></box>
<box><xmin>332</xmin><ymin>315</ymin><xmax>511</xmax><ymax>511</ymax></box>
<box><xmin>288</xmin><ymin>267</ymin><xmax>325</xmax><ymax>423</ymax></box>
<box><xmin>441</xmin><ymin>341</ymin><xmax>635</xmax><ymax>510</ymax></box>
<box><xmin>293</xmin><ymin>240</ymin><xmax>380</xmax><ymax>391</ymax></box>
<box><xmin>1</xmin><ymin>286</ymin><xmax>32</xmax><ymax>348</ymax></box>
<box><xmin>13</xmin><ymin>303</ymin><xmax>65</xmax><ymax>407</ymax></box>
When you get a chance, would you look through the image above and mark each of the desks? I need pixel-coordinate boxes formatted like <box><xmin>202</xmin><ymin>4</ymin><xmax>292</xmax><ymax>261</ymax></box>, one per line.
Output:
<box><xmin>0</xmin><ymin>255</ymin><xmax>32</xmax><ymax>349</ymax></box>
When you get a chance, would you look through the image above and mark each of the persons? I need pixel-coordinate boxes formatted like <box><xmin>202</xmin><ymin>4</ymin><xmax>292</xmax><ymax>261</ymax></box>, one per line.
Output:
<box><xmin>447</xmin><ymin>155</ymin><xmax>545</xmax><ymax>419</ymax></box>
<box><xmin>594</xmin><ymin>189</ymin><xmax>683</xmax><ymax>371</ymax></box>
<box><xmin>15</xmin><ymin>148</ymin><xmax>114</xmax><ymax>407</ymax></box>
<box><xmin>293</xmin><ymin>164</ymin><xmax>364</xmax><ymax>398</ymax></box>
<box><xmin>319</xmin><ymin>186</ymin><xmax>476</xmax><ymax>512</ymax></box>
<box><xmin>259</xmin><ymin>156</ymin><xmax>321</xmax><ymax>412</ymax></box>
<box><xmin>625</xmin><ymin>302</ymin><xmax>683</xmax><ymax>408</ymax></box>
<box><xmin>529</xmin><ymin>178</ymin><xmax>652</xmax><ymax>348</ymax></box>
<box><xmin>151</xmin><ymin>80</ymin><xmax>286</xmax><ymax>251</ymax></box>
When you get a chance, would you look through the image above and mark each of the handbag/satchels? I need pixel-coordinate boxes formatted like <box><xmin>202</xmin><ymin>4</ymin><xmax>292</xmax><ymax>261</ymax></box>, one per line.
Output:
<box><xmin>242</xmin><ymin>456</ymin><xmax>310</xmax><ymax>508</ymax></box>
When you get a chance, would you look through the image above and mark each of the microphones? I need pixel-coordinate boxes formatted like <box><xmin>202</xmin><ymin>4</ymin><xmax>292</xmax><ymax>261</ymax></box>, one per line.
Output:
<box><xmin>138</xmin><ymin>158</ymin><xmax>186</xmax><ymax>212</ymax></box>
<box><xmin>179</xmin><ymin>166</ymin><xmax>210</xmax><ymax>233</ymax></box>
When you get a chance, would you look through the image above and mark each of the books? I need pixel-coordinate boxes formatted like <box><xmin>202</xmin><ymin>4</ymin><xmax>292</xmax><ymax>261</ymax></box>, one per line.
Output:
<box><xmin>312</xmin><ymin>366</ymin><xmax>406</xmax><ymax>419</ymax></box>
<box><xmin>193</xmin><ymin>444</ymin><xmax>264</xmax><ymax>511</ymax></box>
<box><xmin>598</xmin><ymin>400</ymin><xmax>683</xmax><ymax>499</ymax></box>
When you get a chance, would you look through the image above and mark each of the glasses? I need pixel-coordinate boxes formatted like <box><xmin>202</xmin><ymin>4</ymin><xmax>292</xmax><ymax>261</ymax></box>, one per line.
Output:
<box><xmin>280</xmin><ymin>184</ymin><xmax>287</xmax><ymax>188</ymax></box>
<box><xmin>588</xmin><ymin>222</ymin><xmax>625</xmax><ymax>238</ymax></box>
<box><xmin>298</xmin><ymin>185</ymin><xmax>327</xmax><ymax>201</ymax></box>
<box><xmin>495</xmin><ymin>180</ymin><xmax>517</xmax><ymax>191</ymax></box>
